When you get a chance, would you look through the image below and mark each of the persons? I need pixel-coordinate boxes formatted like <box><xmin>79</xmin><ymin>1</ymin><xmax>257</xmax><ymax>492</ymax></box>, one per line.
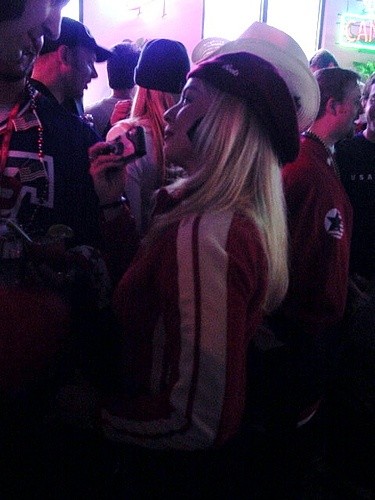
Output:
<box><xmin>57</xmin><ymin>48</ymin><xmax>313</xmax><ymax>500</ymax></box>
<box><xmin>0</xmin><ymin>1</ymin><xmax>374</xmax><ymax>500</ymax></box>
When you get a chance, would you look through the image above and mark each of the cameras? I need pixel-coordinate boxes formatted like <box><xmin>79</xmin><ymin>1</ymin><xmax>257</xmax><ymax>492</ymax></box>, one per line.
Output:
<box><xmin>99</xmin><ymin>126</ymin><xmax>148</xmax><ymax>171</ymax></box>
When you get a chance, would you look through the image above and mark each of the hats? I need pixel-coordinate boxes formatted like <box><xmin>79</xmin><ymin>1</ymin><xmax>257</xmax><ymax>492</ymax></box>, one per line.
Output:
<box><xmin>192</xmin><ymin>21</ymin><xmax>321</xmax><ymax>134</ymax></box>
<box><xmin>133</xmin><ymin>39</ymin><xmax>189</xmax><ymax>95</ymax></box>
<box><xmin>108</xmin><ymin>43</ymin><xmax>139</xmax><ymax>89</ymax></box>
<box><xmin>45</xmin><ymin>16</ymin><xmax>113</xmax><ymax>63</ymax></box>
<box><xmin>186</xmin><ymin>50</ymin><xmax>301</xmax><ymax>164</ymax></box>
